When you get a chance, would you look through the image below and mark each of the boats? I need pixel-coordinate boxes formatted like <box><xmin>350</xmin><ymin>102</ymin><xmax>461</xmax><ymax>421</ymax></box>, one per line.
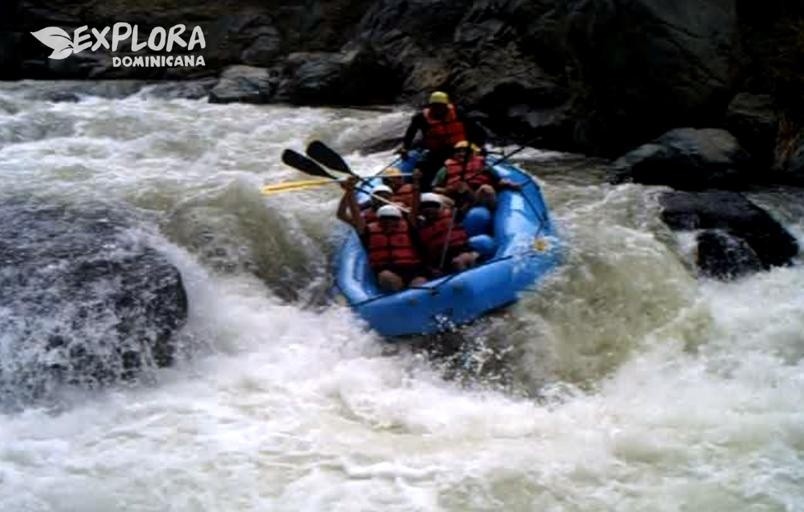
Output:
<box><xmin>328</xmin><ymin>146</ymin><xmax>561</xmax><ymax>340</ymax></box>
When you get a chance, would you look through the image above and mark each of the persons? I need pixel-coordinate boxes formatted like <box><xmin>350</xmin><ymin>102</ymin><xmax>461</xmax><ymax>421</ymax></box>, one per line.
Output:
<box><xmin>394</xmin><ymin>89</ymin><xmax>502</xmax><ymax>187</ymax></box>
<box><xmin>332</xmin><ymin>137</ymin><xmax>521</xmax><ymax>296</ymax></box>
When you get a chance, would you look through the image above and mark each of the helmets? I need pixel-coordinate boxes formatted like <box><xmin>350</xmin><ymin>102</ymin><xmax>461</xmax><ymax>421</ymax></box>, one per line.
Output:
<box><xmin>370</xmin><ymin>184</ymin><xmax>393</xmax><ymax>200</ymax></box>
<box><xmin>418</xmin><ymin>192</ymin><xmax>443</xmax><ymax>210</ymax></box>
<box><xmin>382</xmin><ymin>167</ymin><xmax>402</xmax><ymax>178</ymax></box>
<box><xmin>468</xmin><ymin>235</ymin><xmax>496</xmax><ymax>256</ymax></box>
<box><xmin>429</xmin><ymin>91</ymin><xmax>448</xmax><ymax>105</ymax></box>
<box><xmin>464</xmin><ymin>206</ymin><xmax>491</xmax><ymax>234</ymax></box>
<box><xmin>454</xmin><ymin>140</ymin><xmax>476</xmax><ymax>157</ymax></box>
<box><xmin>376</xmin><ymin>205</ymin><xmax>402</xmax><ymax>219</ymax></box>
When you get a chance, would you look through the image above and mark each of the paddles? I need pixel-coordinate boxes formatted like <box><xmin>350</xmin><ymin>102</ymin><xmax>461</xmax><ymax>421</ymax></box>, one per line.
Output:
<box><xmin>262</xmin><ymin>173</ymin><xmax>414</xmax><ymax>194</ymax></box>
<box><xmin>282</xmin><ymin>149</ymin><xmax>425</xmax><ymax>220</ymax></box>
<box><xmin>306</xmin><ymin>140</ymin><xmax>373</xmax><ymax>187</ymax></box>
